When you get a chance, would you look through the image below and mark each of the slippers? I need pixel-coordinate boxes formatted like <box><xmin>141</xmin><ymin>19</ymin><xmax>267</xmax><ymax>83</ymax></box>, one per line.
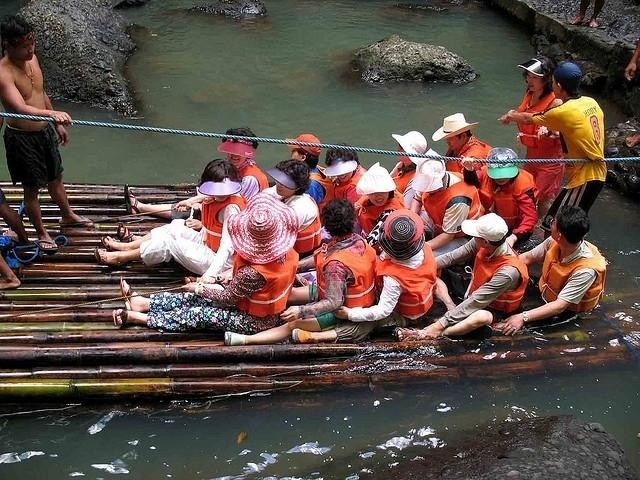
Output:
<box><xmin>36</xmin><ymin>236</ymin><xmax>59</xmax><ymax>253</ymax></box>
<box><xmin>119</xmin><ymin>276</ymin><xmax>135</xmax><ymax>310</ymax></box>
<box><xmin>59</xmin><ymin>213</ymin><xmax>94</xmax><ymax>228</ymax></box>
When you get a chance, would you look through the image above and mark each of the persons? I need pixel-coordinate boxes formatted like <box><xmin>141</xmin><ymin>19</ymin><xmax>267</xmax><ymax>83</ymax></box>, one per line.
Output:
<box><xmin>1</xmin><ymin>188</ymin><xmax>32</xmax><ymax>290</ymax></box>
<box><xmin>623</xmin><ymin>39</ymin><xmax>640</xmax><ymax>149</ymax></box>
<box><xmin>569</xmin><ymin>0</ymin><xmax>606</xmax><ymax>28</ymax></box>
<box><xmin>498</xmin><ymin>56</ymin><xmax>564</xmax><ymax>221</ymax></box>
<box><xmin>503</xmin><ymin>60</ymin><xmax>610</xmax><ymax>239</ymax></box>
<box><xmin>0</xmin><ymin>15</ymin><xmax>95</xmax><ymax>253</ymax></box>
<box><xmin>94</xmin><ymin>114</ymin><xmax>606</xmax><ymax>345</ymax></box>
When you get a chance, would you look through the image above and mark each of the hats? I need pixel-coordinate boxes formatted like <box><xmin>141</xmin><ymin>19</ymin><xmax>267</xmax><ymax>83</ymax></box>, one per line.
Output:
<box><xmin>412</xmin><ymin>157</ymin><xmax>446</xmax><ymax>193</ymax></box>
<box><xmin>391</xmin><ymin>130</ymin><xmax>428</xmax><ymax>165</ymax></box>
<box><xmin>461</xmin><ymin>213</ymin><xmax>509</xmax><ymax>241</ymax></box>
<box><xmin>197</xmin><ymin>178</ymin><xmax>243</xmax><ymax>196</ymax></box>
<box><xmin>485</xmin><ymin>147</ymin><xmax>519</xmax><ymax>180</ymax></box>
<box><xmin>226</xmin><ymin>192</ymin><xmax>299</xmax><ymax>264</ymax></box>
<box><xmin>217</xmin><ymin>138</ymin><xmax>257</xmax><ymax>160</ymax></box>
<box><xmin>432</xmin><ymin>112</ymin><xmax>478</xmax><ymax>142</ymax></box>
<box><xmin>357</xmin><ymin>166</ymin><xmax>397</xmax><ymax>195</ymax></box>
<box><xmin>379</xmin><ymin>210</ymin><xmax>426</xmax><ymax>260</ymax></box>
<box><xmin>264</xmin><ymin>131</ymin><xmax>357</xmax><ymax>189</ymax></box>
<box><xmin>516</xmin><ymin>53</ymin><xmax>582</xmax><ymax>99</ymax></box>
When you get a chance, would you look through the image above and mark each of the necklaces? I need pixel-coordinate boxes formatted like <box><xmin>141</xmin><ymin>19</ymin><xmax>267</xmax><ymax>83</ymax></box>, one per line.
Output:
<box><xmin>20</xmin><ymin>62</ymin><xmax>35</xmax><ymax>87</ymax></box>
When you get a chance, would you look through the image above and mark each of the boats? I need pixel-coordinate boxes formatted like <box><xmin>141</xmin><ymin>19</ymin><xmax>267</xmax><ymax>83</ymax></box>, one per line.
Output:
<box><xmin>1</xmin><ymin>177</ymin><xmax>640</xmax><ymax>400</ymax></box>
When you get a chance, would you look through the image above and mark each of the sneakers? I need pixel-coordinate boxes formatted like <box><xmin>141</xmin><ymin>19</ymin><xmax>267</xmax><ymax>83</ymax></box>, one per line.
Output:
<box><xmin>292</xmin><ymin>327</ymin><xmax>314</xmax><ymax>344</ymax></box>
<box><xmin>224</xmin><ymin>330</ymin><xmax>247</xmax><ymax>347</ymax></box>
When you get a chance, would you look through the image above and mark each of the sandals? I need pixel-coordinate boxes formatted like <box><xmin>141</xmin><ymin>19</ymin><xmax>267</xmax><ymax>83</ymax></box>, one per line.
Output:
<box><xmin>93</xmin><ymin>247</ymin><xmax>125</xmax><ymax>268</ymax></box>
<box><xmin>392</xmin><ymin>326</ymin><xmax>422</xmax><ymax>341</ymax></box>
<box><xmin>100</xmin><ymin>235</ymin><xmax>117</xmax><ymax>249</ymax></box>
<box><xmin>112</xmin><ymin>309</ymin><xmax>127</xmax><ymax>328</ymax></box>
<box><xmin>117</xmin><ymin>222</ymin><xmax>134</xmax><ymax>242</ymax></box>
<box><xmin>122</xmin><ymin>183</ymin><xmax>142</xmax><ymax>215</ymax></box>
